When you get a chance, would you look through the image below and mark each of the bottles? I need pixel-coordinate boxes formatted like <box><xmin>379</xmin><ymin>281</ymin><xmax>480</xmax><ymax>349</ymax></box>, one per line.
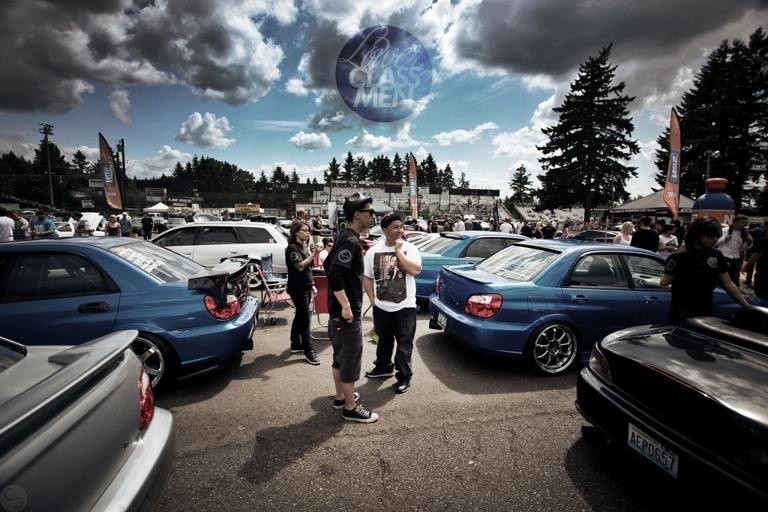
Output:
<box><xmin>691</xmin><ymin>178</ymin><xmax>736</xmax><ymax>229</ymax></box>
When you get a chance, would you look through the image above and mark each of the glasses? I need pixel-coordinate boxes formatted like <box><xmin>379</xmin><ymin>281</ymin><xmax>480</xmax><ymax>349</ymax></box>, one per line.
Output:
<box><xmin>299</xmin><ymin>228</ymin><xmax>310</xmax><ymax>232</ymax></box>
<box><xmin>706</xmin><ymin>232</ymin><xmax>721</xmax><ymax>241</ymax></box>
<box><xmin>358</xmin><ymin>207</ymin><xmax>376</xmax><ymax>216</ymax></box>
<box><xmin>328</xmin><ymin>245</ymin><xmax>333</xmax><ymax>248</ymax></box>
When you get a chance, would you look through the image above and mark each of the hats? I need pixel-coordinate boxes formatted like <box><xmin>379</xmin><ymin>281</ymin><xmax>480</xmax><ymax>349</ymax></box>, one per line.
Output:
<box><xmin>381</xmin><ymin>210</ymin><xmax>402</xmax><ymax>225</ymax></box>
<box><xmin>340</xmin><ymin>192</ymin><xmax>376</xmax><ymax>217</ymax></box>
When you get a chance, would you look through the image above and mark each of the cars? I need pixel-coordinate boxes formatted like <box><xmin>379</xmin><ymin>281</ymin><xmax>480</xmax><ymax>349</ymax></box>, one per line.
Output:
<box><xmin>566</xmin><ymin>298</ymin><xmax>767</xmax><ymax>509</ymax></box>
<box><xmin>568</xmin><ymin>228</ymin><xmax>619</xmax><ymax>244</ymax></box>
<box><xmin>425</xmin><ymin>237</ymin><xmax>759</xmax><ymax>379</ymax></box>
<box><xmin>0</xmin><ymin>234</ymin><xmax>260</xmax><ymax>398</ymax></box>
<box><xmin>0</xmin><ymin>326</ymin><xmax>186</xmax><ymax>512</ymax></box>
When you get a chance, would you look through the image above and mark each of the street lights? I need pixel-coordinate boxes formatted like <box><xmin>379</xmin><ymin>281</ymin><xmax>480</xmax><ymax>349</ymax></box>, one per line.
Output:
<box><xmin>116</xmin><ymin>138</ymin><xmax>128</xmax><ymax>209</ymax></box>
<box><xmin>703</xmin><ymin>149</ymin><xmax>719</xmax><ymax>179</ymax></box>
<box><xmin>39</xmin><ymin>122</ymin><xmax>58</xmax><ymax>207</ymax></box>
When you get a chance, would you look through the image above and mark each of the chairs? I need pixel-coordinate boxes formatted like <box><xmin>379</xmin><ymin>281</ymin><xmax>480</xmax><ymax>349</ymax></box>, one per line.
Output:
<box><xmin>253</xmin><ymin>261</ymin><xmax>292</xmax><ymax>327</ymax></box>
<box><xmin>308</xmin><ymin>273</ymin><xmax>333</xmax><ymax>342</ymax></box>
<box><xmin>260</xmin><ymin>251</ymin><xmax>291</xmax><ymax>307</ymax></box>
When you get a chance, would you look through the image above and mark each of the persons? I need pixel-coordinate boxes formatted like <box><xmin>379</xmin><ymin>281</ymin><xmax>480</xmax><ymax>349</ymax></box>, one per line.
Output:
<box><xmin>1</xmin><ymin>207</ymin><xmax>168</xmax><ymax>243</ymax></box>
<box><xmin>287</xmin><ymin>193</ymin><xmax>765</xmax><ymax>423</ymax></box>
<box><xmin>222</xmin><ymin>210</ymin><xmax>229</xmax><ymax>221</ymax></box>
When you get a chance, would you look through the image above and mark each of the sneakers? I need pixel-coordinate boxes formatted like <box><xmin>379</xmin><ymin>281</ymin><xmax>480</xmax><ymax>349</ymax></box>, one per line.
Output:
<box><xmin>334</xmin><ymin>391</ymin><xmax>360</xmax><ymax>408</ymax></box>
<box><xmin>304</xmin><ymin>346</ymin><xmax>320</xmax><ymax>366</ymax></box>
<box><xmin>365</xmin><ymin>364</ymin><xmax>396</xmax><ymax>378</ymax></box>
<box><xmin>289</xmin><ymin>343</ymin><xmax>309</xmax><ymax>353</ymax></box>
<box><xmin>340</xmin><ymin>405</ymin><xmax>377</xmax><ymax>423</ymax></box>
<box><xmin>396</xmin><ymin>375</ymin><xmax>411</xmax><ymax>392</ymax></box>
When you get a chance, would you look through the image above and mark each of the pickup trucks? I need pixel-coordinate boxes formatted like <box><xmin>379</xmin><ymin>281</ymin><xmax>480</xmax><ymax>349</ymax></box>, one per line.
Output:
<box><xmin>20</xmin><ymin>209</ymin><xmax>108</xmax><ymax>238</ymax></box>
